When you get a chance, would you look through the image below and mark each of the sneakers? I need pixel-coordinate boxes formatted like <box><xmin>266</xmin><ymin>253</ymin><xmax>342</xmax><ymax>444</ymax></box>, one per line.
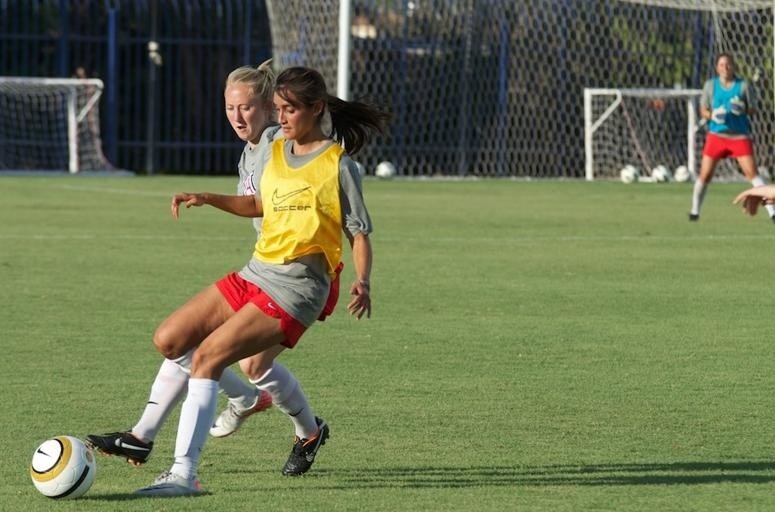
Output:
<box><xmin>135</xmin><ymin>471</ymin><xmax>204</xmax><ymax>495</ymax></box>
<box><xmin>209</xmin><ymin>390</ymin><xmax>272</xmax><ymax>437</ymax></box>
<box><xmin>283</xmin><ymin>417</ymin><xmax>328</xmax><ymax>477</ymax></box>
<box><xmin>689</xmin><ymin>213</ymin><xmax>699</xmax><ymax>219</ymax></box>
<box><xmin>85</xmin><ymin>429</ymin><xmax>154</xmax><ymax>466</ymax></box>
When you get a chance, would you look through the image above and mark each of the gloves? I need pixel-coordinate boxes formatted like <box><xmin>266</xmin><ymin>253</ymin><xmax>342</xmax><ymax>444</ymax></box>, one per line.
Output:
<box><xmin>711</xmin><ymin>106</ymin><xmax>726</xmax><ymax>125</ymax></box>
<box><xmin>730</xmin><ymin>97</ymin><xmax>746</xmax><ymax>116</ymax></box>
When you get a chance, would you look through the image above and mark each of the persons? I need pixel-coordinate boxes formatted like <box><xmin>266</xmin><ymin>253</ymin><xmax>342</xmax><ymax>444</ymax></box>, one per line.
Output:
<box><xmin>688</xmin><ymin>52</ymin><xmax>775</xmax><ymax>224</ymax></box>
<box><xmin>733</xmin><ymin>185</ymin><xmax>775</xmax><ymax>216</ymax></box>
<box><xmin>130</xmin><ymin>67</ymin><xmax>391</xmax><ymax>499</ymax></box>
<box><xmin>85</xmin><ymin>59</ymin><xmax>344</xmax><ymax>477</ymax></box>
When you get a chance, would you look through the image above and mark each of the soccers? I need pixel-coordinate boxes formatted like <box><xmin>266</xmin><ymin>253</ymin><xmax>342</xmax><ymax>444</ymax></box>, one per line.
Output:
<box><xmin>651</xmin><ymin>165</ymin><xmax>670</xmax><ymax>183</ymax></box>
<box><xmin>619</xmin><ymin>164</ymin><xmax>640</xmax><ymax>185</ymax></box>
<box><xmin>29</xmin><ymin>434</ymin><xmax>97</xmax><ymax>501</ymax></box>
<box><xmin>376</xmin><ymin>160</ymin><xmax>396</xmax><ymax>181</ymax></box>
<box><xmin>673</xmin><ymin>165</ymin><xmax>691</xmax><ymax>183</ymax></box>
<box><xmin>353</xmin><ymin>160</ymin><xmax>367</xmax><ymax>179</ymax></box>
